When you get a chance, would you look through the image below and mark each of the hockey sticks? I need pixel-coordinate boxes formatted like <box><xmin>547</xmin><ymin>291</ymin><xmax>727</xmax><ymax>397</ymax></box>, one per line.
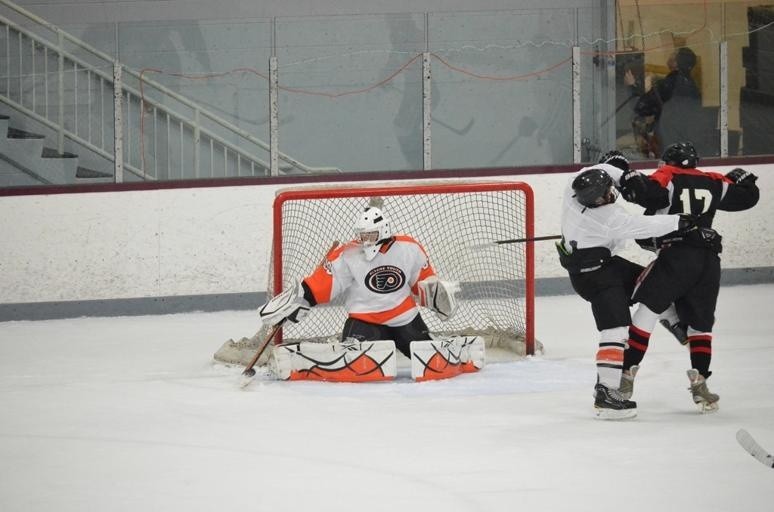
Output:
<box><xmin>464</xmin><ymin>236</ymin><xmax>561</xmax><ymax>251</ymax></box>
<box><xmin>735</xmin><ymin>429</ymin><xmax>774</xmax><ymax>468</ymax></box>
<box><xmin>240</xmin><ymin>240</ymin><xmax>339</xmax><ymax>386</ymax></box>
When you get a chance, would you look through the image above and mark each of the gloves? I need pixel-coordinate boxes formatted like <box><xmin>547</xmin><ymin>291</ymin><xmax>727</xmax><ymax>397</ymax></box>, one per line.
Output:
<box><xmin>725</xmin><ymin>166</ymin><xmax>758</xmax><ymax>184</ymax></box>
<box><xmin>679</xmin><ymin>213</ymin><xmax>708</xmax><ymax>237</ymax></box>
<box><xmin>621</xmin><ymin>170</ymin><xmax>647</xmax><ymax>201</ymax></box>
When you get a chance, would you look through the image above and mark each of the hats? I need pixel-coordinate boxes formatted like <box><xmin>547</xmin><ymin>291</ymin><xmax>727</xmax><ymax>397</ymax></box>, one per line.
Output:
<box><xmin>675</xmin><ymin>48</ymin><xmax>697</xmax><ymax>79</ymax></box>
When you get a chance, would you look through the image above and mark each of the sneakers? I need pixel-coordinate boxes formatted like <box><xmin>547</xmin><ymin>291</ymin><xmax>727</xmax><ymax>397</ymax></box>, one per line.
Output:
<box><xmin>593</xmin><ymin>365</ymin><xmax>640</xmax><ymax>408</ymax></box>
<box><xmin>659</xmin><ymin>319</ymin><xmax>688</xmax><ymax>345</ymax></box>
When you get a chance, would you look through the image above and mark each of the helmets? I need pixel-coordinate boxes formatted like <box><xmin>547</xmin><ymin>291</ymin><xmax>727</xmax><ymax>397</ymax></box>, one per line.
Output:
<box><xmin>572</xmin><ymin>150</ymin><xmax>628</xmax><ymax>208</ymax></box>
<box><xmin>354</xmin><ymin>206</ymin><xmax>390</xmax><ymax>262</ymax></box>
<box><xmin>662</xmin><ymin>142</ymin><xmax>699</xmax><ymax>169</ymax></box>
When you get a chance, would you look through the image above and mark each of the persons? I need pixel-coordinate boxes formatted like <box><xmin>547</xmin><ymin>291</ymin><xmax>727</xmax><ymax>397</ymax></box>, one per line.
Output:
<box><xmin>614</xmin><ymin>143</ymin><xmax>761</xmax><ymax>413</ymax></box>
<box><xmin>561</xmin><ymin>150</ymin><xmax>692</xmax><ymax>413</ymax></box>
<box><xmin>624</xmin><ymin>46</ymin><xmax>699</xmax><ymax>152</ymax></box>
<box><xmin>258</xmin><ymin>204</ymin><xmax>485</xmax><ymax>384</ymax></box>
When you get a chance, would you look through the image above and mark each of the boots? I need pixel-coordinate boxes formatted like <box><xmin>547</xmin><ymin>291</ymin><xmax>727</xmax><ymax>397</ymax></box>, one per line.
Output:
<box><xmin>687</xmin><ymin>369</ymin><xmax>719</xmax><ymax>403</ymax></box>
